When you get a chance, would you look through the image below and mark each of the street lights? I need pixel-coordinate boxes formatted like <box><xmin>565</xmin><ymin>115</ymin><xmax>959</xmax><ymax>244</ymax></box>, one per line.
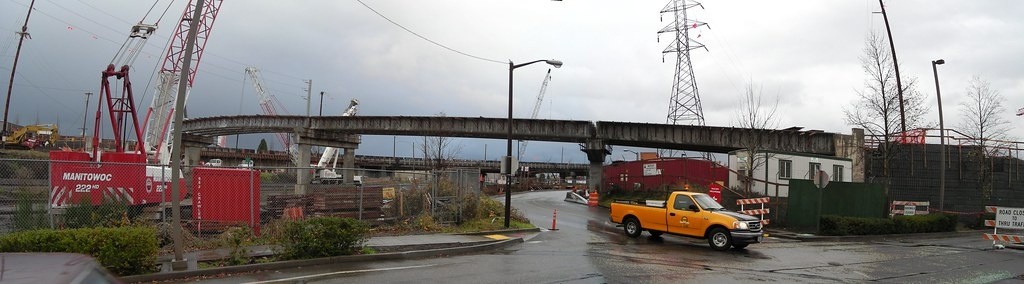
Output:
<box><xmin>504</xmin><ymin>58</ymin><xmax>562</xmax><ymax>226</ymax></box>
<box><xmin>932</xmin><ymin>59</ymin><xmax>946</xmax><ymax>212</ymax></box>
<box><xmin>623</xmin><ymin>149</ymin><xmax>638</xmax><ymax>163</ymax></box>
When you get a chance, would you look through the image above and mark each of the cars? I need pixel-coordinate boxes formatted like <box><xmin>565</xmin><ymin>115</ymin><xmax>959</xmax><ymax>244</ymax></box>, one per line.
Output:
<box><xmin>205</xmin><ymin>159</ymin><xmax>223</xmax><ymax>166</ymax></box>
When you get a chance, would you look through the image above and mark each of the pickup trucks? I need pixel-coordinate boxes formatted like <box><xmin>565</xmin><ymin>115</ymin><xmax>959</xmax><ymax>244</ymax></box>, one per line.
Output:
<box><xmin>611</xmin><ymin>191</ymin><xmax>764</xmax><ymax>251</ymax></box>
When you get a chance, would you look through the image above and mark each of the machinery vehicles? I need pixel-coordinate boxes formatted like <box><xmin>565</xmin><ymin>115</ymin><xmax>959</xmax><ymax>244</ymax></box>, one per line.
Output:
<box><xmin>3</xmin><ymin>124</ymin><xmax>60</xmax><ymax>147</ymax></box>
